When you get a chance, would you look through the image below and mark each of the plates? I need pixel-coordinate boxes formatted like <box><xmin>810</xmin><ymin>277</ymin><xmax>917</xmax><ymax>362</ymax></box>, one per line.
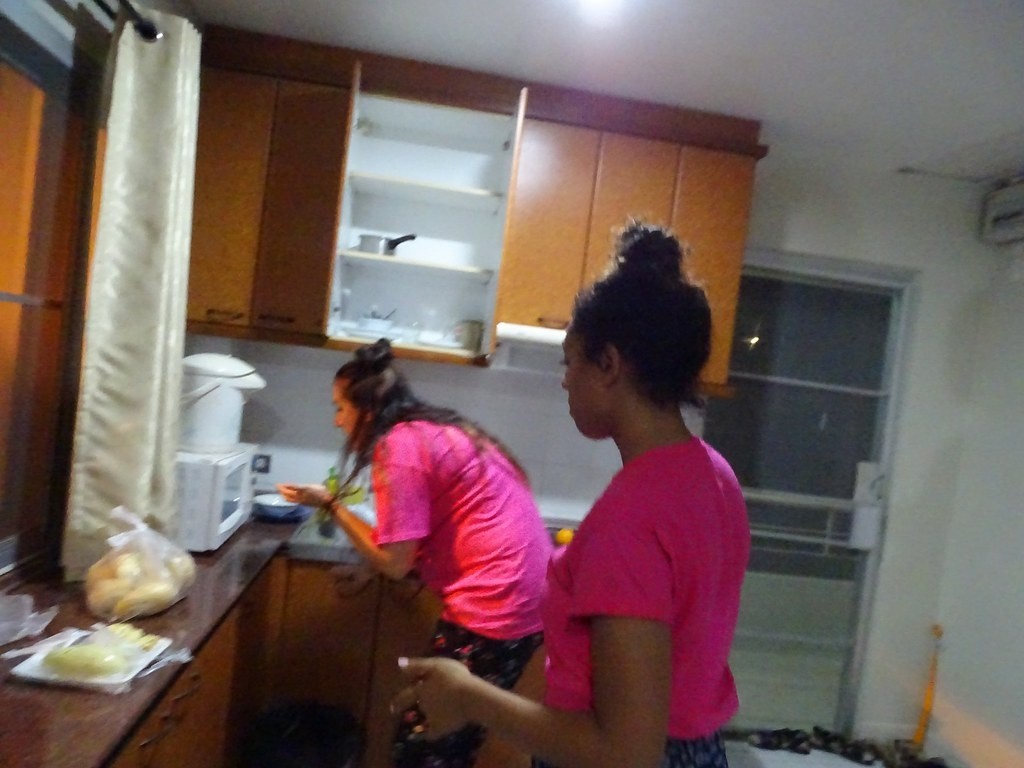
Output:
<box><xmin>340</xmin><ymin>326</ymin><xmax>404</xmax><ymax>340</ymax></box>
<box><xmin>417</xmin><ymin>337</ymin><xmax>463</xmax><ymax>348</ymax></box>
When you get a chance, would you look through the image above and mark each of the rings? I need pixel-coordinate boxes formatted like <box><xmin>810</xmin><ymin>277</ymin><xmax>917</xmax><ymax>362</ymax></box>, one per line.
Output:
<box><xmin>413</xmin><ymin>672</ymin><xmax>426</xmax><ymax>703</ymax></box>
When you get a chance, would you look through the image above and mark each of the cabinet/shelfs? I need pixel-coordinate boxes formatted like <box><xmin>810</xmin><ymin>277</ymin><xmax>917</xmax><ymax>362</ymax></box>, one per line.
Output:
<box><xmin>102</xmin><ymin>553</ymin><xmax>547</xmax><ymax>768</ymax></box>
<box><xmin>186</xmin><ymin>23</ymin><xmax>283</xmax><ymax>339</ymax></box>
<box><xmin>496</xmin><ymin>81</ymin><xmax>768</xmax><ymax>400</ymax></box>
<box><xmin>252</xmin><ymin>39</ymin><xmax>529</xmax><ymax>367</ymax></box>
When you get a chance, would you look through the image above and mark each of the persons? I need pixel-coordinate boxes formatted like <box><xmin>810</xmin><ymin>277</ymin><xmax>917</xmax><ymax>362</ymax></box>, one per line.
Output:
<box><xmin>273</xmin><ymin>337</ymin><xmax>554</xmax><ymax>767</ymax></box>
<box><xmin>390</xmin><ymin>225</ymin><xmax>749</xmax><ymax>768</ymax></box>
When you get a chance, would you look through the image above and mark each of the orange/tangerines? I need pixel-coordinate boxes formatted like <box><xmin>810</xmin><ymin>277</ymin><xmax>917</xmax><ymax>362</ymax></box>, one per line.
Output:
<box><xmin>556</xmin><ymin>530</ymin><xmax>574</xmax><ymax>544</ymax></box>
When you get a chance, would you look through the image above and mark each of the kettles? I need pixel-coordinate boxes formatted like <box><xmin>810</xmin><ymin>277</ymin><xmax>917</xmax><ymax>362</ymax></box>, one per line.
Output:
<box><xmin>177</xmin><ymin>352</ymin><xmax>267</xmax><ymax>454</ymax></box>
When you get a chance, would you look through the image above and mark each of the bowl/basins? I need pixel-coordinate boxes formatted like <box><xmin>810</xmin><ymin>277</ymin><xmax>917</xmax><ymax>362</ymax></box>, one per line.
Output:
<box><xmin>254</xmin><ymin>493</ymin><xmax>316</xmax><ymax>524</ymax></box>
<box><xmin>356</xmin><ymin>317</ymin><xmax>394</xmax><ymax>330</ymax></box>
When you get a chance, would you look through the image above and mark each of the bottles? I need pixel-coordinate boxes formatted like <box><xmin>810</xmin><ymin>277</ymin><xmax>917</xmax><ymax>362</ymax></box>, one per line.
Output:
<box><xmin>342</xmin><ymin>288</ymin><xmax>353</xmax><ymax>321</ymax></box>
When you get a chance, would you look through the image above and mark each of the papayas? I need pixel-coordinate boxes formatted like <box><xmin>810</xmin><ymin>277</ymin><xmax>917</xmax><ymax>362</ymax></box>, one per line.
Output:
<box><xmin>87</xmin><ymin>553</ymin><xmax>196</xmax><ymax>613</ymax></box>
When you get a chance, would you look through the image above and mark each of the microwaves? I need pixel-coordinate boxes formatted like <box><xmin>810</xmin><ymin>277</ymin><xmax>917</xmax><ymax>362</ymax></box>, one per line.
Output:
<box><xmin>177</xmin><ymin>442</ymin><xmax>259</xmax><ymax>552</ymax></box>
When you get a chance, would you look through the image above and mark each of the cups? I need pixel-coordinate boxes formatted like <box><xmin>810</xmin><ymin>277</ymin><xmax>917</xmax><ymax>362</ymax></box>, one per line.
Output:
<box><xmin>461</xmin><ymin>319</ymin><xmax>485</xmax><ymax>351</ymax></box>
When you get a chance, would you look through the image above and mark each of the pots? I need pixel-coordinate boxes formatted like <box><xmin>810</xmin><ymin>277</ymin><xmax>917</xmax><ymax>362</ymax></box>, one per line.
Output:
<box><xmin>357</xmin><ymin>233</ymin><xmax>417</xmax><ymax>256</ymax></box>
